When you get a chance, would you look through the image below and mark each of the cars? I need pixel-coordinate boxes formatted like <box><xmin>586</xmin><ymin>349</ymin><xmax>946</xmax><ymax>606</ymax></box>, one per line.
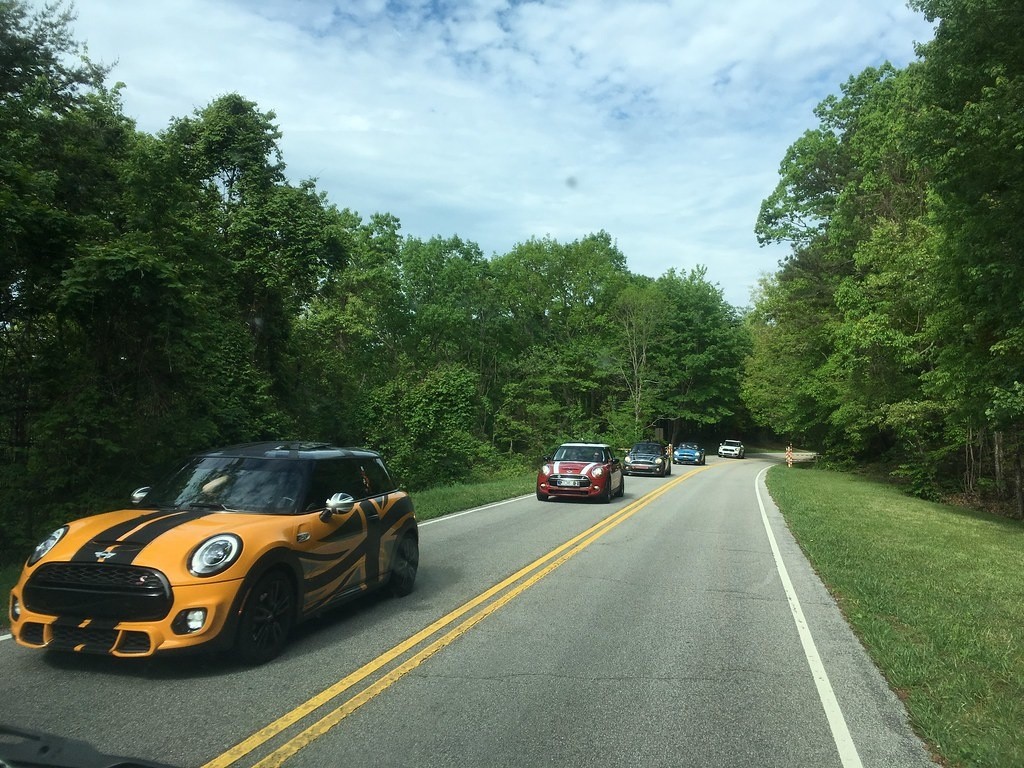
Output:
<box><xmin>718</xmin><ymin>439</ymin><xmax>745</xmax><ymax>459</ymax></box>
<box><xmin>536</xmin><ymin>442</ymin><xmax>625</xmax><ymax>503</ymax></box>
<box><xmin>622</xmin><ymin>443</ymin><xmax>671</xmax><ymax>477</ymax></box>
<box><xmin>673</xmin><ymin>441</ymin><xmax>706</xmax><ymax>466</ymax></box>
<box><xmin>8</xmin><ymin>439</ymin><xmax>420</xmax><ymax>668</ymax></box>
